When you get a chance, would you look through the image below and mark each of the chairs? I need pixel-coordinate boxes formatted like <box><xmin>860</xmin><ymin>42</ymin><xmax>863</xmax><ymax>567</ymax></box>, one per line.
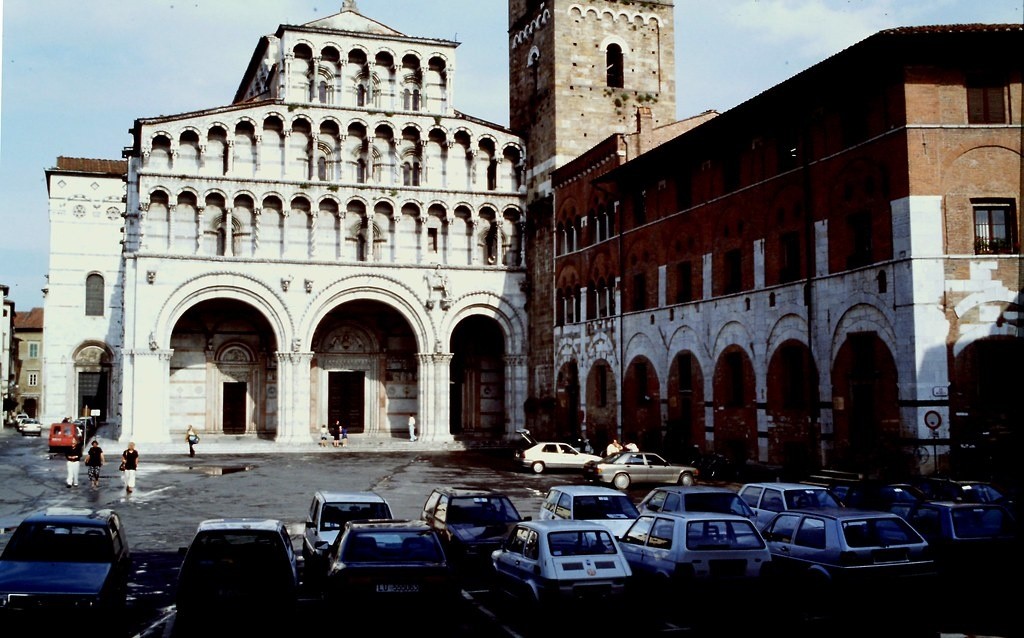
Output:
<box><xmin>845</xmin><ymin>525</ymin><xmax>864</xmax><ymax>539</ymax></box>
<box><xmin>403</xmin><ymin>537</ymin><xmax>435</xmax><ymax>559</ymax></box>
<box><xmin>39</xmin><ymin>530</ymin><xmax>54</xmax><ymax>534</ymax></box>
<box><xmin>450</xmin><ymin>506</ymin><xmax>464</xmax><ymax>523</ymax></box>
<box><xmin>212</xmin><ymin>540</ymin><xmax>231</xmax><ymax>546</ymax></box>
<box><xmin>703</xmin><ymin>526</ymin><xmax>719</xmax><ymax>537</ymax></box>
<box><xmin>85</xmin><ymin>530</ymin><xmax>103</xmax><ymax>535</ymax></box>
<box><xmin>357</xmin><ymin>537</ymin><xmax>376</xmax><ymax>547</ymax></box>
<box><xmin>482</xmin><ymin>502</ymin><xmax>499</xmax><ymax>511</ymax></box>
<box><xmin>590</xmin><ymin>543</ymin><xmax>608</xmax><ymax>552</ymax></box>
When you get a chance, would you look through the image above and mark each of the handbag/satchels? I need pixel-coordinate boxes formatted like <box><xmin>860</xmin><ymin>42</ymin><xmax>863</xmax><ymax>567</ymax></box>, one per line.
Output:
<box><xmin>85</xmin><ymin>459</ymin><xmax>90</xmax><ymax>466</ymax></box>
<box><xmin>189</xmin><ymin>435</ymin><xmax>200</xmax><ymax>444</ymax></box>
<box><xmin>119</xmin><ymin>450</ymin><xmax>127</xmax><ymax>471</ymax></box>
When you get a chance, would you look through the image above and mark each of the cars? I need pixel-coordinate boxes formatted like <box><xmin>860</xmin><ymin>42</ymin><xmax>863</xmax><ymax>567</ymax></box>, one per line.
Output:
<box><xmin>583</xmin><ymin>450</ymin><xmax>699</xmax><ymax>490</ymax></box>
<box><xmin>12</xmin><ymin>413</ymin><xmax>42</xmax><ymax>436</ymax></box>
<box><xmin>65</xmin><ymin>417</ymin><xmax>94</xmax><ymax>444</ymax></box>
<box><xmin>513</xmin><ymin>428</ymin><xmax>604</xmax><ymax>474</ymax></box>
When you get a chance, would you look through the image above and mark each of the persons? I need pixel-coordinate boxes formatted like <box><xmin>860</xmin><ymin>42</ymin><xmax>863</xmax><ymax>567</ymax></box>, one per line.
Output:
<box><xmin>320</xmin><ymin>425</ymin><xmax>329</xmax><ymax>447</ymax></box>
<box><xmin>341</xmin><ymin>427</ymin><xmax>348</xmax><ymax>447</ymax></box>
<box><xmin>121</xmin><ymin>442</ymin><xmax>139</xmax><ymax>494</ymax></box>
<box><xmin>624</xmin><ymin>441</ymin><xmax>640</xmax><ymax>458</ymax></box>
<box><xmin>66</xmin><ymin>439</ymin><xmax>82</xmax><ymax>488</ymax></box>
<box><xmin>185</xmin><ymin>424</ymin><xmax>198</xmax><ymax>457</ymax></box>
<box><xmin>606</xmin><ymin>439</ymin><xmax>623</xmax><ymax>456</ymax></box>
<box><xmin>85</xmin><ymin>440</ymin><xmax>106</xmax><ymax>489</ymax></box>
<box><xmin>333</xmin><ymin>421</ymin><xmax>341</xmax><ymax>447</ymax></box>
<box><xmin>408</xmin><ymin>413</ymin><xmax>418</xmax><ymax>442</ymax></box>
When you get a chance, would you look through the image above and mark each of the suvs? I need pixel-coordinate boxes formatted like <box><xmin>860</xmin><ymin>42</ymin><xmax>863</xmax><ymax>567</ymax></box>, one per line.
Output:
<box><xmin>49</xmin><ymin>423</ymin><xmax>80</xmax><ymax>452</ymax></box>
<box><xmin>635</xmin><ymin>486</ymin><xmax>754</xmax><ymax>532</ymax></box>
<box><xmin>733</xmin><ymin>508</ymin><xmax>930</xmax><ymax>597</ymax></box>
<box><xmin>886</xmin><ymin>499</ymin><xmax>1024</xmax><ymax>570</ymax></box>
<box><xmin>314</xmin><ymin>518</ymin><xmax>448</xmax><ymax>616</ymax></box>
<box><xmin>176</xmin><ymin>518</ymin><xmax>304</xmax><ymax>617</ymax></box>
<box><xmin>302</xmin><ymin>490</ymin><xmax>393</xmax><ymax>576</ymax></box>
<box><xmin>422</xmin><ymin>486</ymin><xmax>533</xmax><ymax>566</ymax></box>
<box><xmin>831</xmin><ymin>475</ymin><xmax>1014</xmax><ymax>516</ymax></box>
<box><xmin>488</xmin><ymin>519</ymin><xmax>633</xmax><ymax>609</ymax></box>
<box><xmin>0</xmin><ymin>507</ymin><xmax>129</xmax><ymax>627</ymax></box>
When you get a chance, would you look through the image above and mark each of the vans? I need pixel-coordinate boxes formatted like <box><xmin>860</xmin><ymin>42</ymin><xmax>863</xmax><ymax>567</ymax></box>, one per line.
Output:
<box><xmin>539</xmin><ymin>484</ymin><xmax>641</xmax><ymax>545</ymax></box>
<box><xmin>729</xmin><ymin>482</ymin><xmax>847</xmax><ymax>536</ymax></box>
<box><xmin>606</xmin><ymin>512</ymin><xmax>771</xmax><ymax>589</ymax></box>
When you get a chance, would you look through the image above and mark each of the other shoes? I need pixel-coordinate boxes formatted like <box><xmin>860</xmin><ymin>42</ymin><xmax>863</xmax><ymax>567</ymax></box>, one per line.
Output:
<box><xmin>126</xmin><ymin>487</ymin><xmax>132</xmax><ymax>493</ymax></box>
<box><xmin>67</xmin><ymin>485</ymin><xmax>71</xmax><ymax>488</ymax></box>
